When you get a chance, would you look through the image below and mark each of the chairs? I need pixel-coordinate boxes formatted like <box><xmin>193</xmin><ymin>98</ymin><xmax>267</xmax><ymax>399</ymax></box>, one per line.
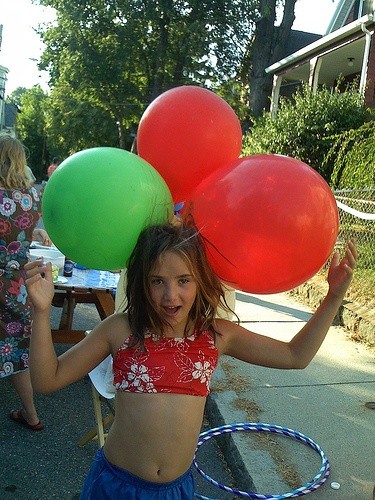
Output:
<box><xmin>80</xmin><ymin>330</ymin><xmax>115</xmax><ymax>448</ymax></box>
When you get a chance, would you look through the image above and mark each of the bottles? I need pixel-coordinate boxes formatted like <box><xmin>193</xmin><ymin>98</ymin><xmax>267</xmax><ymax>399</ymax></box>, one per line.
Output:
<box><xmin>64</xmin><ymin>257</ymin><xmax>73</xmax><ymax>277</ymax></box>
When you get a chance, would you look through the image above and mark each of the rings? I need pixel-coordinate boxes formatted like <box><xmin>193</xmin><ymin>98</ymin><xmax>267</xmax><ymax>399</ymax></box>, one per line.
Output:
<box><xmin>47</xmin><ymin>240</ymin><xmax>49</xmax><ymax>242</ymax></box>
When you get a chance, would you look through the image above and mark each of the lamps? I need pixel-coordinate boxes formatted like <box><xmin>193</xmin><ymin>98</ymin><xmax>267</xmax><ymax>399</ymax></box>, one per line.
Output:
<box><xmin>348</xmin><ymin>58</ymin><xmax>355</xmax><ymax>66</ymax></box>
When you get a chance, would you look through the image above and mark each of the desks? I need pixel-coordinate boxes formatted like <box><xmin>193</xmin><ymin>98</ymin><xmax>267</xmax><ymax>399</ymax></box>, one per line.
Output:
<box><xmin>50</xmin><ymin>268</ymin><xmax>118</xmax><ymax>344</ymax></box>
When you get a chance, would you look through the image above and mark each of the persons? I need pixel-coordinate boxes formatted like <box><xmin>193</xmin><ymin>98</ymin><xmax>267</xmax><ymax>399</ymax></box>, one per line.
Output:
<box><xmin>0</xmin><ymin>134</ymin><xmax>53</xmax><ymax>431</ymax></box>
<box><xmin>23</xmin><ymin>224</ymin><xmax>357</xmax><ymax>500</ymax></box>
<box><xmin>48</xmin><ymin>156</ymin><xmax>58</xmax><ymax>176</ymax></box>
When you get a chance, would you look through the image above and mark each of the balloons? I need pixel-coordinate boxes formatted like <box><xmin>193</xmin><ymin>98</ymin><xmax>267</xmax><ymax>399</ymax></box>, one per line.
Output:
<box><xmin>188</xmin><ymin>155</ymin><xmax>338</xmax><ymax>295</ymax></box>
<box><xmin>136</xmin><ymin>85</ymin><xmax>242</xmax><ymax>204</ymax></box>
<box><xmin>41</xmin><ymin>148</ymin><xmax>176</xmax><ymax>272</ymax></box>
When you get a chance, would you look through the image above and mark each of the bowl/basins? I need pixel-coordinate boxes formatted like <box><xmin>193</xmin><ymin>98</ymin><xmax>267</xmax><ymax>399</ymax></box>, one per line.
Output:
<box><xmin>29</xmin><ymin>248</ymin><xmax>66</xmax><ymax>270</ymax></box>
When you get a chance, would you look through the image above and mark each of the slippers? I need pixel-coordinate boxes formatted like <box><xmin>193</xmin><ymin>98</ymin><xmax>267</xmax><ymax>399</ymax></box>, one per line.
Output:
<box><xmin>11</xmin><ymin>409</ymin><xmax>42</xmax><ymax>429</ymax></box>
